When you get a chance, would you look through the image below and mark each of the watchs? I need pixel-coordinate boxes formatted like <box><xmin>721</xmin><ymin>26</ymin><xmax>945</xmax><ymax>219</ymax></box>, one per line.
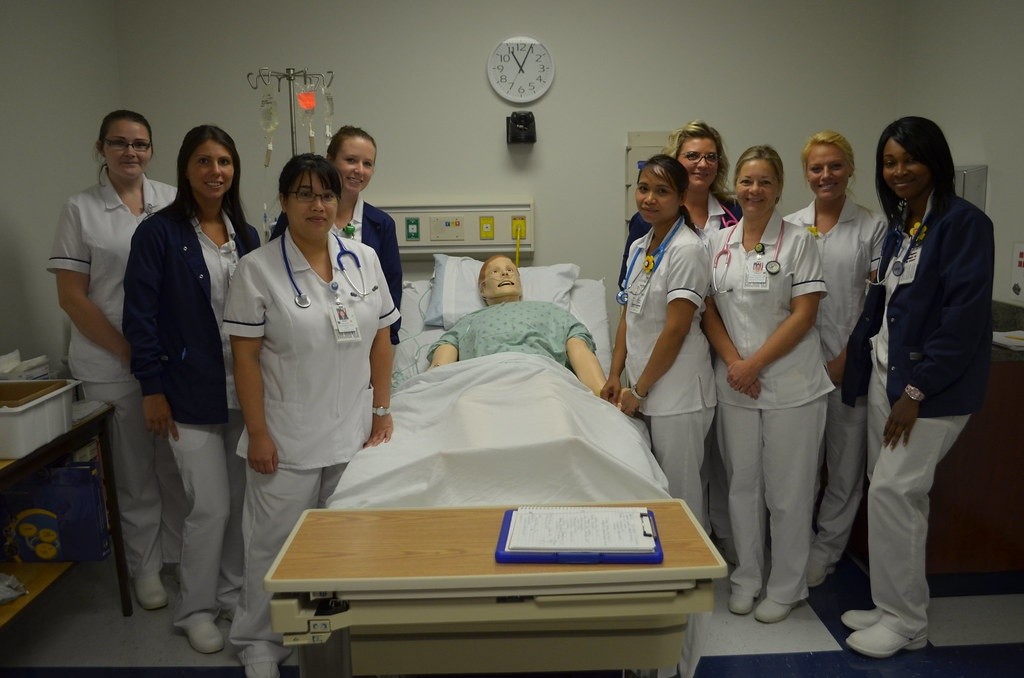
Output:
<box><xmin>905</xmin><ymin>385</ymin><xmax>925</xmax><ymax>401</ymax></box>
<box><xmin>373</xmin><ymin>406</ymin><xmax>391</xmax><ymax>416</ymax></box>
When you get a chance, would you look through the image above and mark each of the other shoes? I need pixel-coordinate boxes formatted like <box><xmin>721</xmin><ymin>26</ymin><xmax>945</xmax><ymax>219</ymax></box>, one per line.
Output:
<box><xmin>841</xmin><ymin>608</ymin><xmax>882</xmax><ymax>629</ymax></box>
<box><xmin>719</xmin><ymin>537</ymin><xmax>739</xmax><ymax>565</ymax></box>
<box><xmin>219</xmin><ymin>605</ymin><xmax>236</xmax><ymax>620</ymax></box>
<box><xmin>186</xmin><ymin>622</ymin><xmax>224</xmax><ymax>654</ymax></box>
<box><xmin>728</xmin><ymin>594</ymin><xmax>755</xmax><ymax>614</ymax></box>
<box><xmin>805</xmin><ymin>560</ymin><xmax>837</xmax><ymax>585</ymax></box>
<box><xmin>754</xmin><ymin>599</ymin><xmax>798</xmax><ymax>622</ymax></box>
<box><xmin>133</xmin><ymin>573</ymin><xmax>168</xmax><ymax>610</ymax></box>
<box><xmin>846</xmin><ymin>622</ymin><xmax>928</xmax><ymax>658</ymax></box>
<box><xmin>245</xmin><ymin>661</ymin><xmax>281</xmax><ymax>678</ymax></box>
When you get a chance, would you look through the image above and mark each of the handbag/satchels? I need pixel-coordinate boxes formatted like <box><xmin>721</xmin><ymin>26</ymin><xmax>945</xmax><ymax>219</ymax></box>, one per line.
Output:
<box><xmin>1</xmin><ymin>458</ymin><xmax>112</xmax><ymax>563</ymax></box>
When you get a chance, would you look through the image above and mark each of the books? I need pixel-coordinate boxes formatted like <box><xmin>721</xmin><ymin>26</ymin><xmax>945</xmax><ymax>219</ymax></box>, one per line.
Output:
<box><xmin>504</xmin><ymin>506</ymin><xmax>656</xmax><ymax>553</ymax></box>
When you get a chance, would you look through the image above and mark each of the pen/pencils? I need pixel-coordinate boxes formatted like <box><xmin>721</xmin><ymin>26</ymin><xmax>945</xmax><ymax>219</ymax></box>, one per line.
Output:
<box><xmin>1003</xmin><ymin>335</ymin><xmax>1024</xmax><ymax>340</ymax></box>
<box><xmin>182</xmin><ymin>348</ymin><xmax>185</xmax><ymax>360</ymax></box>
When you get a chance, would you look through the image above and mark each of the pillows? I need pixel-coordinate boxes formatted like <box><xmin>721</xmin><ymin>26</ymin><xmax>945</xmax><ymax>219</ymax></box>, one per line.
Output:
<box><xmin>424</xmin><ymin>254</ymin><xmax>581</xmax><ymax>330</ymax></box>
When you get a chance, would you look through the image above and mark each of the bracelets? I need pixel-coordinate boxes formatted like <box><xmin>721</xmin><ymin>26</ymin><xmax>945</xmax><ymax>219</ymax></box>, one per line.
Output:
<box><xmin>632</xmin><ymin>385</ymin><xmax>649</xmax><ymax>400</ymax></box>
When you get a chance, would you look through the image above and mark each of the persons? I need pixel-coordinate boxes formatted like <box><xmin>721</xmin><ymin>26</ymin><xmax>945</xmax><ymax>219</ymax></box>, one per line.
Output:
<box><xmin>122</xmin><ymin>125</ymin><xmax>262</xmax><ymax>654</ymax></box>
<box><xmin>601</xmin><ymin>154</ymin><xmax>716</xmax><ymax>536</ymax></box>
<box><xmin>619</xmin><ymin>121</ymin><xmax>742</xmax><ymax>564</ymax></box>
<box><xmin>426</xmin><ymin>255</ymin><xmax>630</xmax><ymax>408</ymax></box>
<box><xmin>339</xmin><ymin>310</ymin><xmax>349</xmax><ymax>320</ymax></box>
<box><xmin>267</xmin><ymin>125</ymin><xmax>403</xmax><ymax>345</ymax></box>
<box><xmin>48</xmin><ymin>109</ymin><xmax>183</xmax><ymax>610</ymax></box>
<box><xmin>222</xmin><ymin>153</ymin><xmax>400</xmax><ymax>678</ymax></box>
<box><xmin>700</xmin><ymin>145</ymin><xmax>835</xmax><ymax>621</ymax></box>
<box><xmin>755</xmin><ymin>264</ymin><xmax>761</xmax><ymax>271</ymax></box>
<box><xmin>782</xmin><ymin>131</ymin><xmax>887</xmax><ymax>588</ymax></box>
<box><xmin>841</xmin><ymin>116</ymin><xmax>995</xmax><ymax>658</ymax></box>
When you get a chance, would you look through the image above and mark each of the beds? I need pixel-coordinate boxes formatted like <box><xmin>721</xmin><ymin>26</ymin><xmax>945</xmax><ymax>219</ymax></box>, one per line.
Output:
<box><xmin>265</xmin><ymin>278</ymin><xmax>731</xmax><ymax>678</ymax></box>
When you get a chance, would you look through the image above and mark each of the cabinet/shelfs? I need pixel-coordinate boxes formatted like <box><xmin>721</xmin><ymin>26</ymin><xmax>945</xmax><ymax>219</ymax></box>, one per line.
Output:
<box><xmin>849</xmin><ymin>357</ymin><xmax>1024</xmax><ymax>573</ymax></box>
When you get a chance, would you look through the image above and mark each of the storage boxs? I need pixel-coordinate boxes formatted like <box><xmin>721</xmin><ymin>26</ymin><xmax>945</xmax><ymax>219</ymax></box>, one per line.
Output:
<box><xmin>1</xmin><ymin>365</ymin><xmax>84</xmax><ymax>461</ymax></box>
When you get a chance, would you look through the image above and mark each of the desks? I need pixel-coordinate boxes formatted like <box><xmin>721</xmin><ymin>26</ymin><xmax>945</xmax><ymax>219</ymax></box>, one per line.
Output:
<box><xmin>0</xmin><ymin>400</ymin><xmax>133</xmax><ymax>628</ymax></box>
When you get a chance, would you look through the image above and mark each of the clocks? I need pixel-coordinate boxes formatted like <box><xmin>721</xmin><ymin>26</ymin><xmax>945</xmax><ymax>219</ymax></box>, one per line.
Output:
<box><xmin>487</xmin><ymin>36</ymin><xmax>555</xmax><ymax>104</ymax></box>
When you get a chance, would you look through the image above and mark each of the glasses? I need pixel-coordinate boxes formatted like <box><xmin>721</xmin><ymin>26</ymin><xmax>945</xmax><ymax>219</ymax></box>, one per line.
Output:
<box><xmin>679</xmin><ymin>151</ymin><xmax>722</xmax><ymax>163</ymax></box>
<box><xmin>287</xmin><ymin>190</ymin><xmax>338</xmax><ymax>203</ymax></box>
<box><xmin>104</xmin><ymin>138</ymin><xmax>151</xmax><ymax>151</ymax></box>
